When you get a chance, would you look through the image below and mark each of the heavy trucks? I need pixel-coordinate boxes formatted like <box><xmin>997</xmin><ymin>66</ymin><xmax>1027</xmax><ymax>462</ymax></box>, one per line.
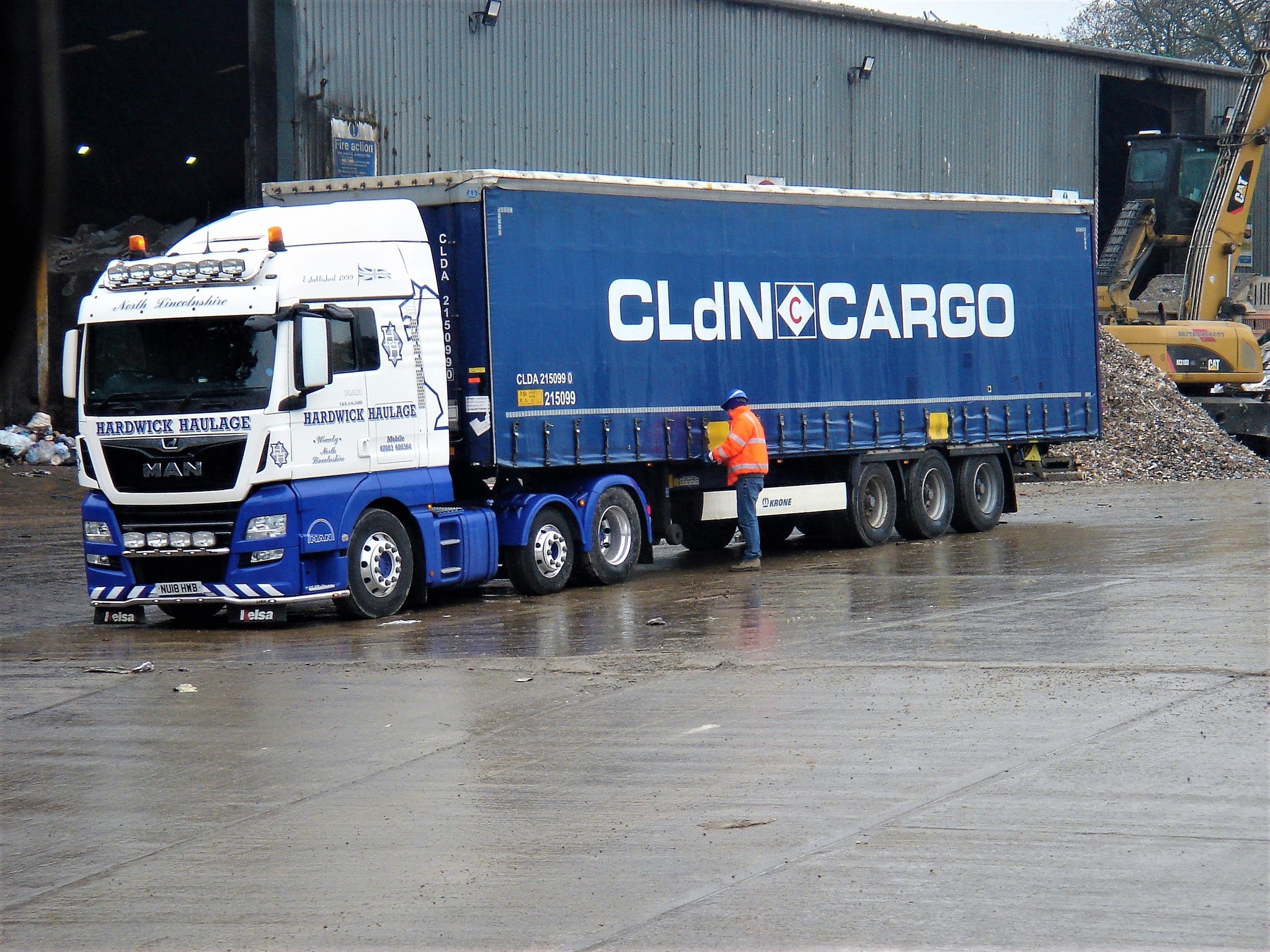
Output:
<box><xmin>62</xmin><ymin>170</ymin><xmax>1101</xmax><ymax>624</ymax></box>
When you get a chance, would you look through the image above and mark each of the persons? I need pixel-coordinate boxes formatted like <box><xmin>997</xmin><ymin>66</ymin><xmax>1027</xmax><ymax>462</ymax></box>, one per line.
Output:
<box><xmin>697</xmin><ymin>389</ymin><xmax>769</xmax><ymax>572</ymax></box>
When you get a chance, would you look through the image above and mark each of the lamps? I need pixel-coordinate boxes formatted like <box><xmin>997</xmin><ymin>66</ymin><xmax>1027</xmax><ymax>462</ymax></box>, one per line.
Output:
<box><xmin>469</xmin><ymin>1</ymin><xmax>501</xmax><ymax>32</ymax></box>
<box><xmin>848</xmin><ymin>53</ymin><xmax>877</xmax><ymax>86</ymax></box>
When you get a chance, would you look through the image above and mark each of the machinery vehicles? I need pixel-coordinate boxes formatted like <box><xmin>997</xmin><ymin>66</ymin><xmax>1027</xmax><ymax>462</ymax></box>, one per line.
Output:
<box><xmin>1099</xmin><ymin>24</ymin><xmax>1270</xmax><ymax>393</ymax></box>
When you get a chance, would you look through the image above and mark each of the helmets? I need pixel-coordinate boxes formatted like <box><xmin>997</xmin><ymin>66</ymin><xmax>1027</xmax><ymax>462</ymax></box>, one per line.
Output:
<box><xmin>720</xmin><ymin>388</ymin><xmax>747</xmax><ymax>410</ymax></box>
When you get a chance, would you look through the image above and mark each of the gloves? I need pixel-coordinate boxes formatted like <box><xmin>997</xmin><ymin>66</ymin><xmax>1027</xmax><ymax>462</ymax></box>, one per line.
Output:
<box><xmin>702</xmin><ymin>451</ymin><xmax>716</xmax><ymax>463</ymax></box>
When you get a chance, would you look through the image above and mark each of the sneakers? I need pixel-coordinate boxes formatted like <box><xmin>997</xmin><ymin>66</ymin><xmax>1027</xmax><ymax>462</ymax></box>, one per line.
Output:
<box><xmin>730</xmin><ymin>558</ymin><xmax>760</xmax><ymax>572</ymax></box>
